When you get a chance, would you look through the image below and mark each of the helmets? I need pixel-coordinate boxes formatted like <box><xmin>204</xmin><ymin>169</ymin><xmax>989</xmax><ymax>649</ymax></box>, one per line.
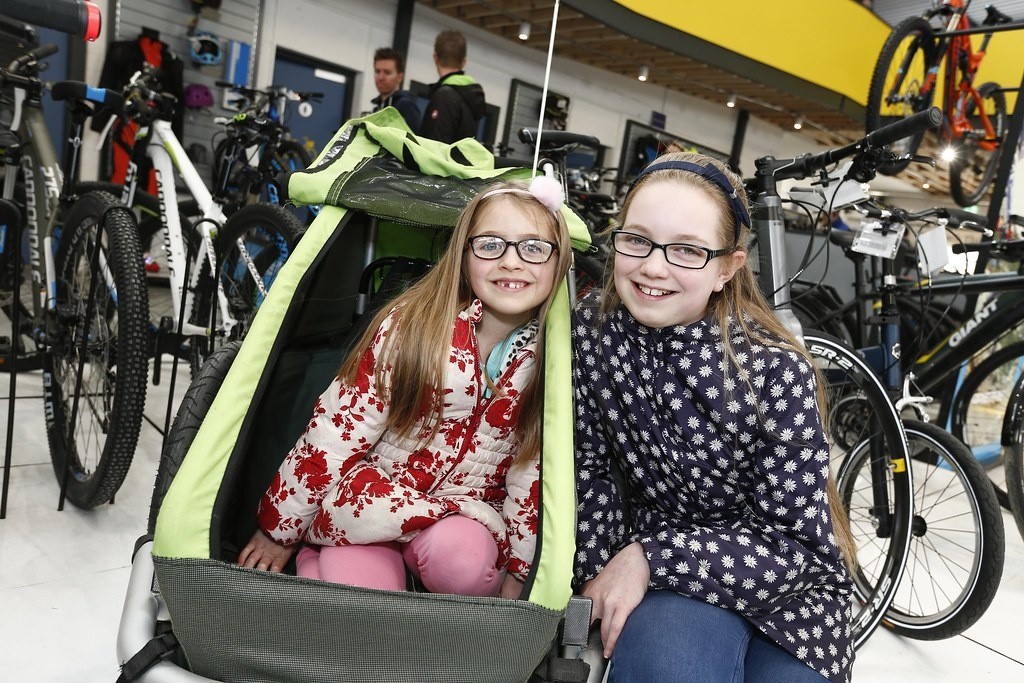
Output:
<box><xmin>183</xmin><ymin>83</ymin><xmax>213</xmax><ymax>108</ymax></box>
<box><xmin>190</xmin><ymin>30</ymin><xmax>222</xmax><ymax>65</ymax></box>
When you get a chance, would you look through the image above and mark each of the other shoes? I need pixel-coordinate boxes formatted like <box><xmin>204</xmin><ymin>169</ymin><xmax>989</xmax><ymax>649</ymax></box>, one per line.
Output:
<box><xmin>143</xmin><ymin>256</ymin><xmax>160</xmax><ymax>273</ymax></box>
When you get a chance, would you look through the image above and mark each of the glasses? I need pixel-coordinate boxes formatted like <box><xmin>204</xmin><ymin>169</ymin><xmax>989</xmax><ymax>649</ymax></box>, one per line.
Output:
<box><xmin>611</xmin><ymin>228</ymin><xmax>738</xmax><ymax>270</ymax></box>
<box><xmin>466</xmin><ymin>235</ymin><xmax>558</xmax><ymax>264</ymax></box>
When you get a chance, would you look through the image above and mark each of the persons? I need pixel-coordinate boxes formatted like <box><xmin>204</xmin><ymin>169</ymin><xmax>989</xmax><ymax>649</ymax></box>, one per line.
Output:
<box><xmin>572</xmin><ymin>152</ymin><xmax>855</xmax><ymax>683</ymax></box>
<box><xmin>236</xmin><ymin>175</ymin><xmax>572</xmax><ymax>599</ymax></box>
<box><xmin>370</xmin><ymin>47</ymin><xmax>421</xmax><ymax>135</ymax></box>
<box><xmin>417</xmin><ymin>30</ymin><xmax>485</xmax><ymax>144</ymax></box>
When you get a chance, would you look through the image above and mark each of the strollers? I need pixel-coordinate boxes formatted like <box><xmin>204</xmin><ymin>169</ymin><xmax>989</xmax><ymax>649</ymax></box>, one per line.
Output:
<box><xmin>117</xmin><ymin>105</ymin><xmax>592</xmax><ymax>683</ymax></box>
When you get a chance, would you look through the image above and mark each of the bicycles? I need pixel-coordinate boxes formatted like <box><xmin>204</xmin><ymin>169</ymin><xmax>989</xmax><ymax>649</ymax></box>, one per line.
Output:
<box><xmin>866</xmin><ymin>0</ymin><xmax>1012</xmax><ymax>208</ymax></box>
<box><xmin>0</xmin><ymin>45</ymin><xmax>150</xmax><ymax>509</ymax></box>
<box><xmin>96</xmin><ymin>67</ymin><xmax>1024</xmax><ymax>641</ymax></box>
